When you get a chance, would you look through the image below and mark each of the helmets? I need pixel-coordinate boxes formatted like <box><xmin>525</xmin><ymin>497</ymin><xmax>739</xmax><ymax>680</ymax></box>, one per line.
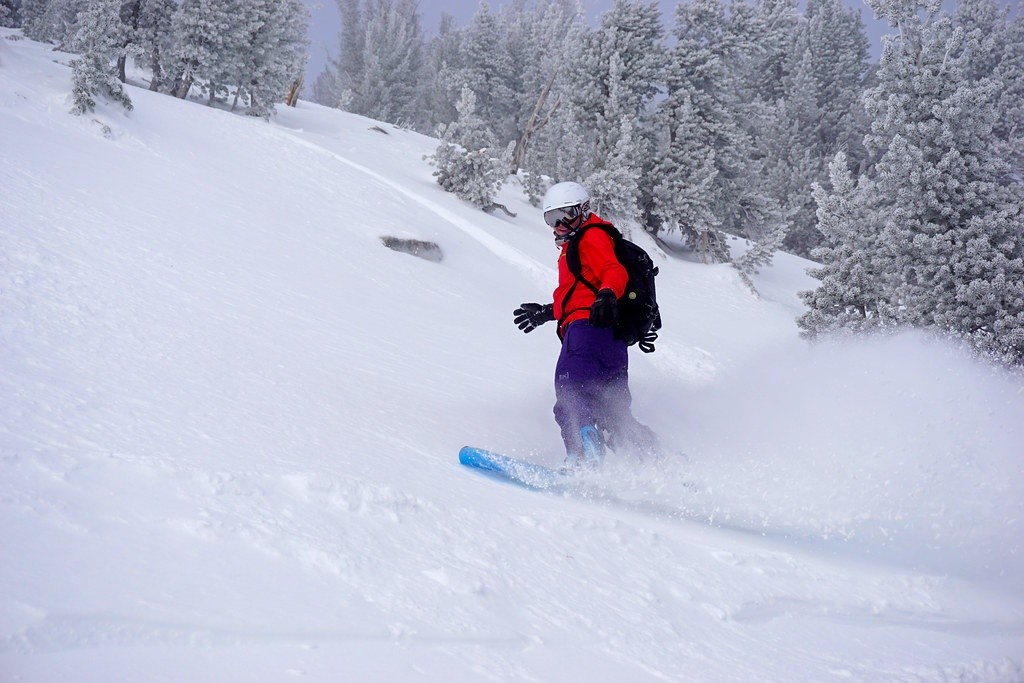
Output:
<box><xmin>543</xmin><ymin>181</ymin><xmax>590</xmax><ymax>213</ymax></box>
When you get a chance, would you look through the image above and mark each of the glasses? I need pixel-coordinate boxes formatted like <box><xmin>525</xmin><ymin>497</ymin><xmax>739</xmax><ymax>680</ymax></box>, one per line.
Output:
<box><xmin>544</xmin><ymin>200</ymin><xmax>589</xmax><ymax>227</ymax></box>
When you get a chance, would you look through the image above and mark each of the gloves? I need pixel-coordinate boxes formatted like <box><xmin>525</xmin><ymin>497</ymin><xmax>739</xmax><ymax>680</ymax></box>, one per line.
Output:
<box><xmin>513</xmin><ymin>302</ymin><xmax>557</xmax><ymax>333</ymax></box>
<box><xmin>588</xmin><ymin>288</ymin><xmax>617</xmax><ymax>329</ymax></box>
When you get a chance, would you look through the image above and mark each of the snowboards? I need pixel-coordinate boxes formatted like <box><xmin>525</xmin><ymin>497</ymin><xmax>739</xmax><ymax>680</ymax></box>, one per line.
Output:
<box><xmin>456</xmin><ymin>443</ymin><xmax>831</xmax><ymax>545</ymax></box>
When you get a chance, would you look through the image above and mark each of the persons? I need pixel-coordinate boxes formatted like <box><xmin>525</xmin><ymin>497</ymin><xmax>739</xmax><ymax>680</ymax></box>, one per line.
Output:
<box><xmin>514</xmin><ymin>182</ymin><xmax>693</xmax><ymax>477</ymax></box>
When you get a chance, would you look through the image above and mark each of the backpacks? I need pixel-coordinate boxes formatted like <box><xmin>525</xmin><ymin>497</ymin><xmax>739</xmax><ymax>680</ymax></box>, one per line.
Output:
<box><xmin>565</xmin><ymin>222</ymin><xmax>662</xmax><ymax>346</ymax></box>
